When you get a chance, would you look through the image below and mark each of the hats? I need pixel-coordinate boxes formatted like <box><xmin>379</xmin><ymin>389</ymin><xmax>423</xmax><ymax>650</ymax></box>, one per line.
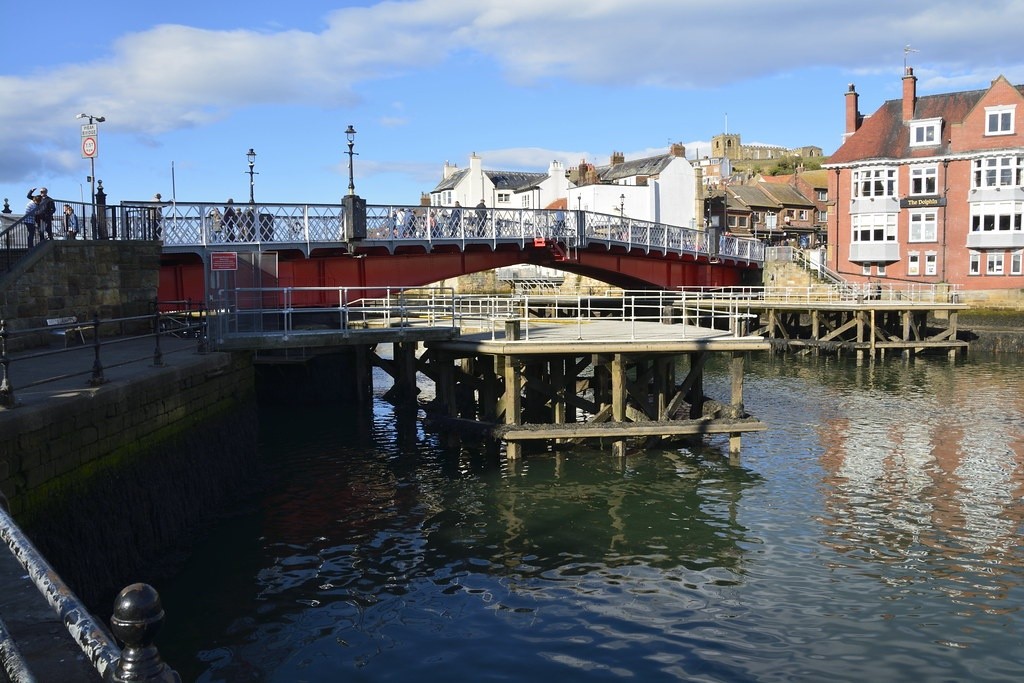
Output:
<box><xmin>455</xmin><ymin>201</ymin><xmax>459</xmax><ymax>203</ymax></box>
<box><xmin>481</xmin><ymin>199</ymin><xmax>485</xmax><ymax>202</ymax></box>
<box><xmin>236</xmin><ymin>208</ymin><xmax>241</xmax><ymax>211</ymax></box>
<box><xmin>154</xmin><ymin>194</ymin><xmax>161</xmax><ymax>199</ymax></box>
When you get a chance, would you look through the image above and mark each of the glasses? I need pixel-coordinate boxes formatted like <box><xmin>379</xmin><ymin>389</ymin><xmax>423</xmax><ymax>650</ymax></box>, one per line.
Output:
<box><xmin>40</xmin><ymin>190</ymin><xmax>46</xmax><ymax>194</ymax></box>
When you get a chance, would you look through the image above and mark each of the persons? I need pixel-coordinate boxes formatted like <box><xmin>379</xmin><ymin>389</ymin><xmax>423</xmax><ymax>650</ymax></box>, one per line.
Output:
<box><xmin>387</xmin><ymin>206</ymin><xmax>417</xmax><ymax>238</ymax></box>
<box><xmin>63</xmin><ymin>204</ymin><xmax>80</xmax><ymax>241</ymax></box>
<box><xmin>208</xmin><ymin>199</ymin><xmax>249</xmax><ymax>245</ymax></box>
<box><xmin>428</xmin><ymin>201</ymin><xmax>450</xmax><ymax>238</ymax></box>
<box><xmin>451</xmin><ymin>201</ymin><xmax>465</xmax><ymax>238</ymax></box>
<box><xmin>150</xmin><ymin>192</ymin><xmax>173</xmax><ymax>242</ymax></box>
<box><xmin>555</xmin><ymin>205</ymin><xmax>566</xmax><ymax>237</ymax></box>
<box><xmin>609</xmin><ymin>225</ymin><xmax>708</xmax><ymax>252</ymax></box>
<box><xmin>475</xmin><ymin>199</ymin><xmax>487</xmax><ymax>238</ymax></box>
<box><xmin>24</xmin><ymin>187</ymin><xmax>55</xmax><ymax>250</ymax></box>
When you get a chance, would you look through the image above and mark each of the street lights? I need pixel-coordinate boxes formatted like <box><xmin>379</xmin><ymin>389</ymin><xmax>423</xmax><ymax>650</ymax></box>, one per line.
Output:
<box><xmin>619</xmin><ymin>194</ymin><xmax>626</xmax><ymax>238</ymax></box>
<box><xmin>345</xmin><ymin>124</ymin><xmax>358</xmax><ymax>197</ymax></box>
<box><xmin>244</xmin><ymin>147</ymin><xmax>259</xmax><ymax>203</ymax></box>
<box><xmin>76</xmin><ymin>111</ymin><xmax>106</xmax><ymax>238</ymax></box>
